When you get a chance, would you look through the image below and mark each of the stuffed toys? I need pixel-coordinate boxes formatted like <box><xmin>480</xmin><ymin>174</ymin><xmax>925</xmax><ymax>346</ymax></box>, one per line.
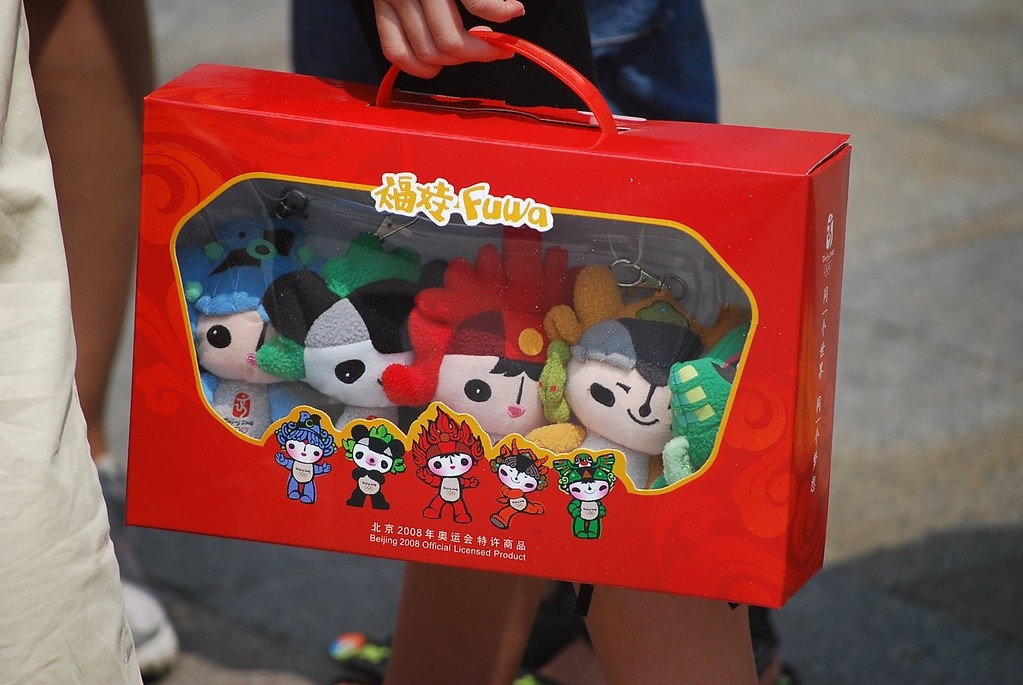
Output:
<box><xmin>178</xmin><ymin>210</ymin><xmax>754</xmax><ymax>490</ymax></box>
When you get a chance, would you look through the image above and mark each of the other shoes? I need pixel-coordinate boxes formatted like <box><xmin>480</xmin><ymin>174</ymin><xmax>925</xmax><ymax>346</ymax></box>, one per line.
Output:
<box><xmin>121</xmin><ymin>580</ymin><xmax>179</xmax><ymax>677</ymax></box>
<box><xmin>331</xmin><ymin>576</ymin><xmax>580</xmax><ymax>683</ymax></box>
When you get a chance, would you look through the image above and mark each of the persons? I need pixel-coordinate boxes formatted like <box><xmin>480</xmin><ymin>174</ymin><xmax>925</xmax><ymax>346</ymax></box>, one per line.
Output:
<box><xmin>289</xmin><ymin>0</ymin><xmax>800</xmax><ymax>684</ymax></box>
<box><xmin>0</xmin><ymin>0</ymin><xmax>178</xmax><ymax>685</ymax></box>
